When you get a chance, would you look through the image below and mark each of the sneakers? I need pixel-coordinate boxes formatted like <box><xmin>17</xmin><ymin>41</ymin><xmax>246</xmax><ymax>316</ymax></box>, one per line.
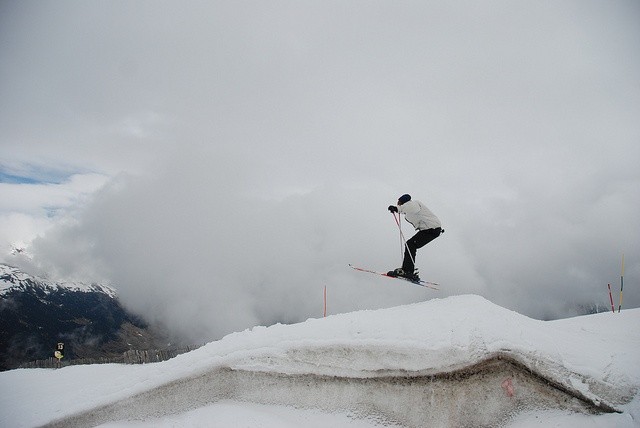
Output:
<box><xmin>394</xmin><ymin>268</ymin><xmax>413</xmax><ymax>279</ymax></box>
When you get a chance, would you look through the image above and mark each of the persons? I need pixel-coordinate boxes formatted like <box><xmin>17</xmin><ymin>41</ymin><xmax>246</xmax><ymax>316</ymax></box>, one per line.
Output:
<box><xmin>388</xmin><ymin>193</ymin><xmax>442</xmax><ymax>278</ymax></box>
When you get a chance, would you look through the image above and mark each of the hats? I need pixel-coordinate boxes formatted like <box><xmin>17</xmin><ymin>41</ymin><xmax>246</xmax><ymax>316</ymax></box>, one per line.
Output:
<box><xmin>398</xmin><ymin>194</ymin><xmax>411</xmax><ymax>205</ymax></box>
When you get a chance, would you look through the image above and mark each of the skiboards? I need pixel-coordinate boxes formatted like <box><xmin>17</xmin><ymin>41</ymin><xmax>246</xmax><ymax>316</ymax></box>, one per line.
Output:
<box><xmin>348</xmin><ymin>262</ymin><xmax>440</xmax><ymax>291</ymax></box>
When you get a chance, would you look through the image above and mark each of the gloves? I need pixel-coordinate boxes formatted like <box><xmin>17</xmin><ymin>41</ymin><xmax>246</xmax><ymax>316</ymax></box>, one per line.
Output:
<box><xmin>388</xmin><ymin>205</ymin><xmax>398</xmax><ymax>213</ymax></box>
